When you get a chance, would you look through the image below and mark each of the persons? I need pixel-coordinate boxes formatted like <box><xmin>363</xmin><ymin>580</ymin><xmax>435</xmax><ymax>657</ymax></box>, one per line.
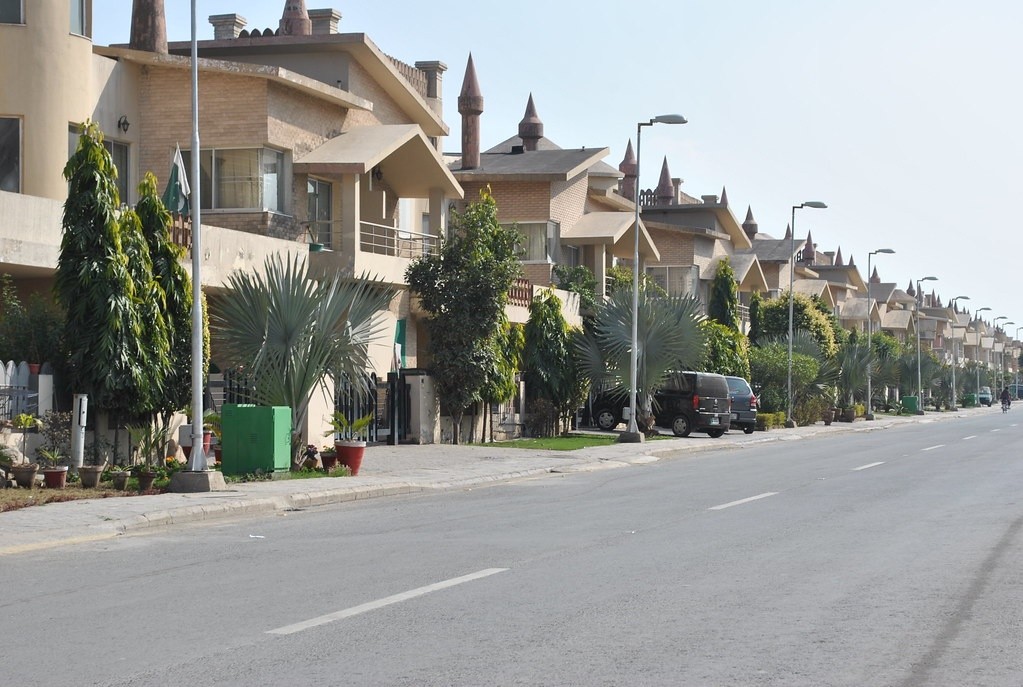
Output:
<box><xmin>1000</xmin><ymin>387</ymin><xmax>1012</xmax><ymax>409</ymax></box>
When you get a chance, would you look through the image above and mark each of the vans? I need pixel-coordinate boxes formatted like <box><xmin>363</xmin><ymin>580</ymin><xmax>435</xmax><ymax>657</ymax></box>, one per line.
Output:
<box><xmin>592</xmin><ymin>370</ymin><xmax>730</xmax><ymax>438</ymax></box>
<box><xmin>979</xmin><ymin>386</ymin><xmax>993</xmax><ymax>407</ymax></box>
<box><xmin>1007</xmin><ymin>383</ymin><xmax>1023</xmax><ymax>399</ymax></box>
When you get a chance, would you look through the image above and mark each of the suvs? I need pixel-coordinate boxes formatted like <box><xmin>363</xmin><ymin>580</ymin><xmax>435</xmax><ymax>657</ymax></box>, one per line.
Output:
<box><xmin>724</xmin><ymin>376</ymin><xmax>757</xmax><ymax>435</ymax></box>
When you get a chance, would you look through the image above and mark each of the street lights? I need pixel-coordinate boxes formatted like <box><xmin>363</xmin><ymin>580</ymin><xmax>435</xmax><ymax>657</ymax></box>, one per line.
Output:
<box><xmin>917</xmin><ymin>276</ymin><xmax>938</xmax><ymax>416</ymax></box>
<box><xmin>617</xmin><ymin>112</ymin><xmax>691</xmax><ymax>444</ymax></box>
<box><xmin>949</xmin><ymin>295</ymin><xmax>970</xmax><ymax>411</ymax></box>
<box><xmin>866</xmin><ymin>247</ymin><xmax>896</xmax><ymax>423</ymax></box>
<box><xmin>992</xmin><ymin>315</ymin><xmax>1007</xmax><ymax>404</ymax></box>
<box><xmin>1001</xmin><ymin>322</ymin><xmax>1016</xmax><ymax>393</ymax></box>
<box><xmin>1015</xmin><ymin>326</ymin><xmax>1023</xmax><ymax>400</ymax></box>
<box><xmin>786</xmin><ymin>201</ymin><xmax>826</xmax><ymax>428</ymax></box>
<box><xmin>974</xmin><ymin>307</ymin><xmax>993</xmax><ymax>407</ymax></box>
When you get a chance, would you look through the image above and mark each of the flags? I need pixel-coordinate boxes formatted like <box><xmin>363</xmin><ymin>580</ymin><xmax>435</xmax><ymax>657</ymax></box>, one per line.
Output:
<box><xmin>161</xmin><ymin>150</ymin><xmax>190</xmax><ymax>220</ymax></box>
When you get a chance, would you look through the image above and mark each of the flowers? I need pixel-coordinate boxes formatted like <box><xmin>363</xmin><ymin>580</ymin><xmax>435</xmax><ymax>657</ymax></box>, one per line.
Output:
<box><xmin>303</xmin><ymin>445</ymin><xmax>318</xmax><ymax>460</ymax></box>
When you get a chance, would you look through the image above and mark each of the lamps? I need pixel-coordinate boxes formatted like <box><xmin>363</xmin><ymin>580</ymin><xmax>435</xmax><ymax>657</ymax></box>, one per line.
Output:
<box><xmin>373</xmin><ymin>166</ymin><xmax>383</xmax><ymax>181</ymax></box>
<box><xmin>118</xmin><ymin>115</ymin><xmax>130</xmax><ymax>134</ymax></box>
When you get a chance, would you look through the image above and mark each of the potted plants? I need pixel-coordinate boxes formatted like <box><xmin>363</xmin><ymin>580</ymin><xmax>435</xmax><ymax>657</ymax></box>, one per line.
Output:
<box><xmin>10</xmin><ymin>413</ymin><xmax>42</xmax><ymax>489</ymax></box>
<box><xmin>40</xmin><ymin>446</ymin><xmax>68</xmax><ymax>489</ymax></box>
<box><xmin>124</xmin><ymin>421</ymin><xmax>171</xmax><ymax>490</ymax></box>
<box><xmin>320</xmin><ymin>411</ymin><xmax>373</xmax><ymax>476</ymax></box>
<box><xmin>178</xmin><ymin>405</ymin><xmax>222</xmax><ymax>461</ymax></box>
<box><xmin>110</xmin><ymin>465</ymin><xmax>133</xmax><ymax>490</ymax></box>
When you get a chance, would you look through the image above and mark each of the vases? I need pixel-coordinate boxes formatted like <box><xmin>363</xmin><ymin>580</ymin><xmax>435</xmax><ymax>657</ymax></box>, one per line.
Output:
<box><xmin>29</xmin><ymin>364</ymin><xmax>39</xmax><ymax>374</ymax></box>
<box><xmin>77</xmin><ymin>465</ymin><xmax>103</xmax><ymax>488</ymax></box>
<box><xmin>844</xmin><ymin>409</ymin><xmax>856</xmax><ymax>420</ymax></box>
<box><xmin>822</xmin><ymin>408</ymin><xmax>842</xmax><ymax>425</ymax></box>
<box><xmin>306</xmin><ymin>459</ymin><xmax>318</xmax><ymax>468</ymax></box>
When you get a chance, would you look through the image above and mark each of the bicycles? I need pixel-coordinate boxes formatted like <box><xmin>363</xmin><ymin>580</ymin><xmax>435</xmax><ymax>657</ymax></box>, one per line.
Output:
<box><xmin>998</xmin><ymin>397</ymin><xmax>1011</xmax><ymax>413</ymax></box>
